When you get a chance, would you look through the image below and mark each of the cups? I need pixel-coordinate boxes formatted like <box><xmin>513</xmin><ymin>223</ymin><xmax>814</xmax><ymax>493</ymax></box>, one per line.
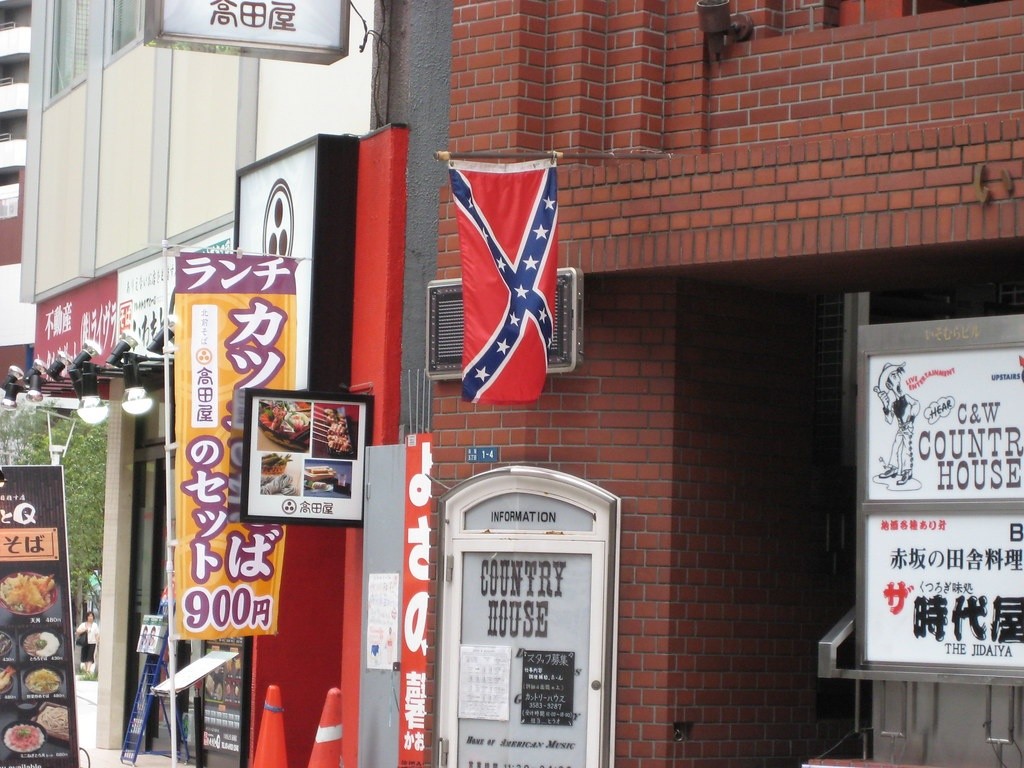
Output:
<box><xmin>338</xmin><ymin>474</ymin><xmax>347</xmax><ymax>487</ymax></box>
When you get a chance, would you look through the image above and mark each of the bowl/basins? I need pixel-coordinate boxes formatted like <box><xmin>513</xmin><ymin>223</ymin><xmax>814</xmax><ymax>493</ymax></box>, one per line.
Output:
<box><xmin>0</xmin><ymin>571</ymin><xmax>58</xmax><ymax>617</ymax></box>
<box><xmin>0</xmin><ymin>668</ymin><xmax>13</xmax><ymax>698</ymax></box>
<box><xmin>23</xmin><ymin>664</ymin><xmax>64</xmax><ymax>694</ymax></box>
<box><xmin>1</xmin><ymin>720</ymin><xmax>47</xmax><ymax>755</ymax></box>
<box><xmin>20</xmin><ymin>627</ymin><xmax>63</xmax><ymax>658</ymax></box>
<box><xmin>0</xmin><ymin>631</ymin><xmax>13</xmax><ymax>659</ymax></box>
<box><xmin>15</xmin><ymin>700</ymin><xmax>38</xmax><ymax>715</ymax></box>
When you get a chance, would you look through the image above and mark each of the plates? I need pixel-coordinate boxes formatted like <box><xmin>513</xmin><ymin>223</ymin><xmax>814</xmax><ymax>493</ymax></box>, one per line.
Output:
<box><xmin>31</xmin><ymin>701</ymin><xmax>69</xmax><ymax>742</ymax></box>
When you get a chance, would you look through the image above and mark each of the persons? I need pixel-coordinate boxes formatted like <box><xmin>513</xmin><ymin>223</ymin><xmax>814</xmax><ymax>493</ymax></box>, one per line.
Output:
<box><xmin>75</xmin><ymin>612</ymin><xmax>98</xmax><ymax>673</ymax></box>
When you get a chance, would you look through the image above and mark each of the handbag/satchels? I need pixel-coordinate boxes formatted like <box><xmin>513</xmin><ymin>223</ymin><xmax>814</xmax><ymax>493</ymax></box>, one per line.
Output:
<box><xmin>76</xmin><ymin>631</ymin><xmax>88</xmax><ymax>647</ymax></box>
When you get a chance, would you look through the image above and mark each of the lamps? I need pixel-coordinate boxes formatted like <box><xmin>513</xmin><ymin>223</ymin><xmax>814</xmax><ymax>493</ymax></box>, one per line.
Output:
<box><xmin>0</xmin><ymin>326</ymin><xmax>174</xmax><ymax>425</ymax></box>
<box><xmin>697</xmin><ymin>0</ymin><xmax>752</xmax><ymax>62</ymax></box>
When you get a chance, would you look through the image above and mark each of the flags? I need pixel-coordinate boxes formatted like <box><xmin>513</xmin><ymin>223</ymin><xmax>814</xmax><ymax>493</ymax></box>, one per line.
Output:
<box><xmin>447</xmin><ymin>160</ymin><xmax>558</xmax><ymax>405</ymax></box>
<box><xmin>174</xmin><ymin>254</ymin><xmax>299</xmax><ymax>639</ymax></box>
